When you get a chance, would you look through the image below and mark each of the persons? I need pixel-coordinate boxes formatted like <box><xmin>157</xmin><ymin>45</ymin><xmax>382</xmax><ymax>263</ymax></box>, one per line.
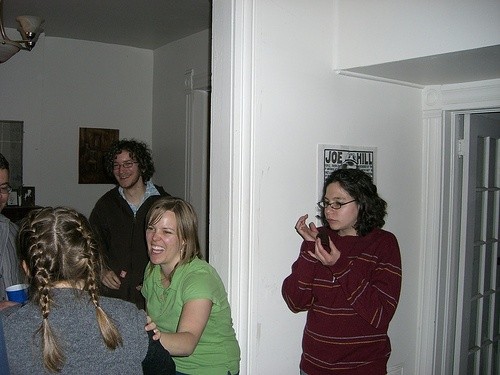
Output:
<box><xmin>0</xmin><ymin>207</ymin><xmax>175</xmax><ymax>375</ymax></box>
<box><xmin>141</xmin><ymin>197</ymin><xmax>241</xmax><ymax>375</ymax></box>
<box><xmin>88</xmin><ymin>139</ymin><xmax>172</xmax><ymax>311</ymax></box>
<box><xmin>0</xmin><ymin>153</ymin><xmax>29</xmax><ymax>308</ymax></box>
<box><xmin>281</xmin><ymin>169</ymin><xmax>402</xmax><ymax>375</ymax></box>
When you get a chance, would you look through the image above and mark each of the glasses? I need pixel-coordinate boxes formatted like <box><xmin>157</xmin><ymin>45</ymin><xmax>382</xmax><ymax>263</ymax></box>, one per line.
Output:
<box><xmin>111</xmin><ymin>160</ymin><xmax>138</xmax><ymax>170</ymax></box>
<box><xmin>0</xmin><ymin>184</ymin><xmax>12</xmax><ymax>194</ymax></box>
<box><xmin>319</xmin><ymin>200</ymin><xmax>356</xmax><ymax>210</ymax></box>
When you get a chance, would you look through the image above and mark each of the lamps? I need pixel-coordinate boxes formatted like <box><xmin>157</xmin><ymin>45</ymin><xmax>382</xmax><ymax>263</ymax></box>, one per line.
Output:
<box><xmin>0</xmin><ymin>0</ymin><xmax>46</xmax><ymax>63</ymax></box>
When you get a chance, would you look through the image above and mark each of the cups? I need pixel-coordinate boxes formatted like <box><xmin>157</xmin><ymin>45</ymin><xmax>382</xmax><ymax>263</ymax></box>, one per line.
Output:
<box><xmin>5</xmin><ymin>284</ymin><xmax>32</xmax><ymax>306</ymax></box>
